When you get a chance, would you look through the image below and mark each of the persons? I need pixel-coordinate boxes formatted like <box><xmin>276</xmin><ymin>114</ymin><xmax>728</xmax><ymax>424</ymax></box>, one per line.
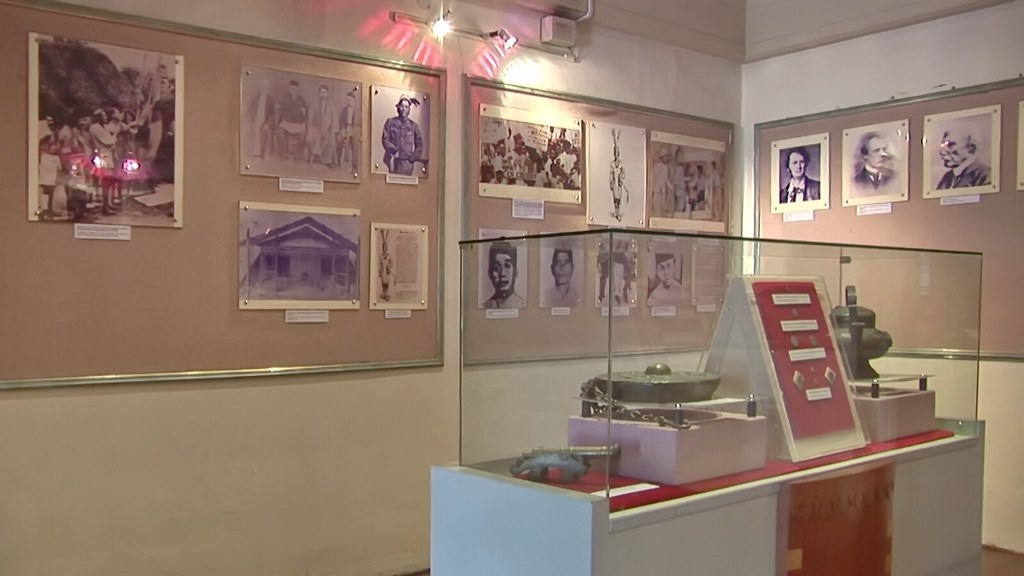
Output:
<box><xmin>246</xmin><ymin>79</ymin><xmax>358</xmax><ymax>175</ymax></box>
<box><xmin>480</xmin><ymin>126</ymin><xmax>581</xmax><ymax>190</ymax></box>
<box><xmin>382</xmin><ymin>93</ymin><xmax>423</xmax><ymax>176</ymax></box>
<box><xmin>652</xmin><ymin>145</ymin><xmax>724</xmax><ymax>222</ymax></box>
<box><xmin>38</xmin><ymin>78</ymin><xmax>176</xmax><ymax>218</ymax></box>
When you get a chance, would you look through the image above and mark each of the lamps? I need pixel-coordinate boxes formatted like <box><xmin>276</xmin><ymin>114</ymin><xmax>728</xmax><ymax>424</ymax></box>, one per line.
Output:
<box><xmin>495</xmin><ymin>29</ymin><xmax>518</xmax><ymax>48</ymax></box>
